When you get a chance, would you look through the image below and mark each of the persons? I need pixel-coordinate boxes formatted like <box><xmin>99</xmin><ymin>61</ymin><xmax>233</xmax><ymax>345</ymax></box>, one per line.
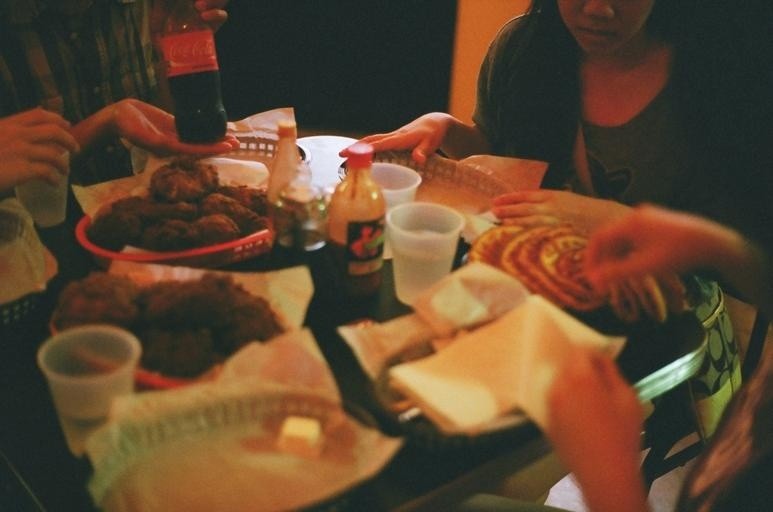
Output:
<box><xmin>339</xmin><ymin>0</ymin><xmax>773</xmax><ymax>302</ymax></box>
<box><xmin>453</xmin><ymin>204</ymin><xmax>773</xmax><ymax>512</ymax></box>
<box><xmin>0</xmin><ymin>0</ymin><xmax>238</xmax><ymax>188</ymax></box>
<box><xmin>1</xmin><ymin>103</ymin><xmax>238</xmax><ymax>187</ymax></box>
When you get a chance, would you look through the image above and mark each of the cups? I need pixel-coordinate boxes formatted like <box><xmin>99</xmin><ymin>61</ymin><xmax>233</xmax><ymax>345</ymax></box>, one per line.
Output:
<box><xmin>33</xmin><ymin>324</ymin><xmax>142</xmax><ymax>459</ymax></box>
<box><xmin>369</xmin><ymin>159</ymin><xmax>421</xmax><ymax>259</ymax></box>
<box><xmin>389</xmin><ymin>200</ymin><xmax>461</xmax><ymax>308</ymax></box>
<box><xmin>12</xmin><ymin>150</ymin><xmax>72</xmax><ymax>228</ymax></box>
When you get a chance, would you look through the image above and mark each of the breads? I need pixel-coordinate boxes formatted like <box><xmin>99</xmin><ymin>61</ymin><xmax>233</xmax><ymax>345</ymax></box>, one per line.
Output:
<box><xmin>389</xmin><ymin>296</ymin><xmax>629</xmax><ymax>441</ymax></box>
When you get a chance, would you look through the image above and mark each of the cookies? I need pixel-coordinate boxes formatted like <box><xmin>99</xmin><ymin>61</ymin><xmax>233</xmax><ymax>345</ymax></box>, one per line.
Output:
<box><xmin>53</xmin><ymin>273</ymin><xmax>278</xmax><ymax>364</ymax></box>
<box><xmin>88</xmin><ymin>156</ymin><xmax>268</xmax><ymax>250</ymax></box>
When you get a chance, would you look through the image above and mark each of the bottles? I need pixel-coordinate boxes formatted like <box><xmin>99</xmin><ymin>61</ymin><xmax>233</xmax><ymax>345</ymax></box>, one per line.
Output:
<box><xmin>266</xmin><ymin>124</ymin><xmax>302</xmax><ymax>238</ymax></box>
<box><xmin>326</xmin><ymin>145</ymin><xmax>385</xmax><ymax>316</ymax></box>
<box><xmin>277</xmin><ymin>168</ymin><xmax>329</xmax><ymax>254</ymax></box>
<box><xmin>162</xmin><ymin>3</ymin><xmax>230</xmax><ymax>143</ymax></box>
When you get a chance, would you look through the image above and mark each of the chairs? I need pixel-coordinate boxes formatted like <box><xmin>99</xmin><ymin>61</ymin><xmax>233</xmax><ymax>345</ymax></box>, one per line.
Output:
<box><xmin>642</xmin><ymin>271</ymin><xmax>771</xmax><ymax>500</ymax></box>
